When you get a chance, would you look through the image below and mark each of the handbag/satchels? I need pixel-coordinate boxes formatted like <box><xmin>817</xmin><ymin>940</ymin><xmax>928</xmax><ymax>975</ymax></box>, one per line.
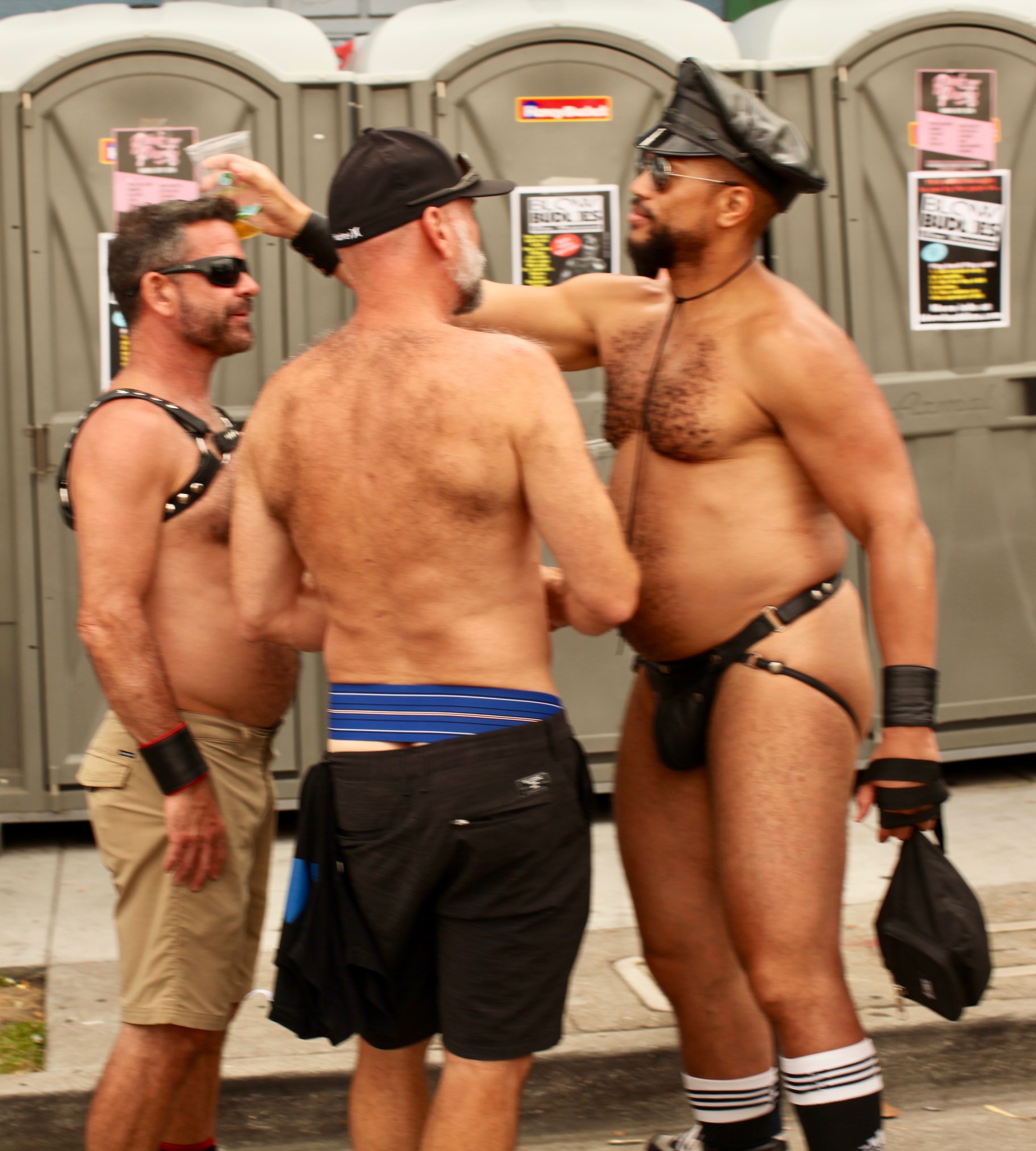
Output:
<box><xmin>874</xmin><ymin>832</ymin><xmax>993</xmax><ymax>1019</ymax></box>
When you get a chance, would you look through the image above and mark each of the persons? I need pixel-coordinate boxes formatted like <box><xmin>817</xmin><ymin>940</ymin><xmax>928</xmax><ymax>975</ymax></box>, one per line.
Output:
<box><xmin>194</xmin><ymin>55</ymin><xmax>950</xmax><ymax>1151</ymax></box>
<box><xmin>57</xmin><ymin>195</ymin><xmax>319</xmax><ymax>1151</ymax></box>
<box><xmin>227</xmin><ymin>129</ymin><xmax>638</xmax><ymax>1151</ymax></box>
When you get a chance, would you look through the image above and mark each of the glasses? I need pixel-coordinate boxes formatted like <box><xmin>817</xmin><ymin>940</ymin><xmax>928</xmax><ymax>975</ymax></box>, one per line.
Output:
<box><xmin>161</xmin><ymin>257</ymin><xmax>246</xmax><ymax>288</ymax></box>
<box><xmin>635</xmin><ymin>153</ymin><xmax>740</xmax><ymax>195</ymax></box>
<box><xmin>403</xmin><ymin>151</ymin><xmax>481</xmax><ymax>207</ymax></box>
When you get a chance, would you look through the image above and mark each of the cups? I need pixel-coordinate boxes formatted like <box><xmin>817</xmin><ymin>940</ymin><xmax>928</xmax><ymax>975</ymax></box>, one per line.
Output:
<box><xmin>187</xmin><ymin>131</ymin><xmax>265</xmax><ymax>241</ymax></box>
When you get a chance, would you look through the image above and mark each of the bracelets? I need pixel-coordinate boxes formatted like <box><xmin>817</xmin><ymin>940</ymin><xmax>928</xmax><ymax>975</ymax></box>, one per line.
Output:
<box><xmin>882</xmin><ymin>662</ymin><xmax>937</xmax><ymax>727</ymax></box>
<box><xmin>135</xmin><ymin>721</ymin><xmax>208</xmax><ymax>797</ymax></box>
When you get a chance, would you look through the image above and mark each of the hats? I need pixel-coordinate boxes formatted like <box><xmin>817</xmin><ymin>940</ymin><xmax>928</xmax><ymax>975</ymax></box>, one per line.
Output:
<box><xmin>634</xmin><ymin>59</ymin><xmax>826</xmax><ymax>212</ymax></box>
<box><xmin>331</xmin><ymin>128</ymin><xmax>518</xmax><ymax>248</ymax></box>
